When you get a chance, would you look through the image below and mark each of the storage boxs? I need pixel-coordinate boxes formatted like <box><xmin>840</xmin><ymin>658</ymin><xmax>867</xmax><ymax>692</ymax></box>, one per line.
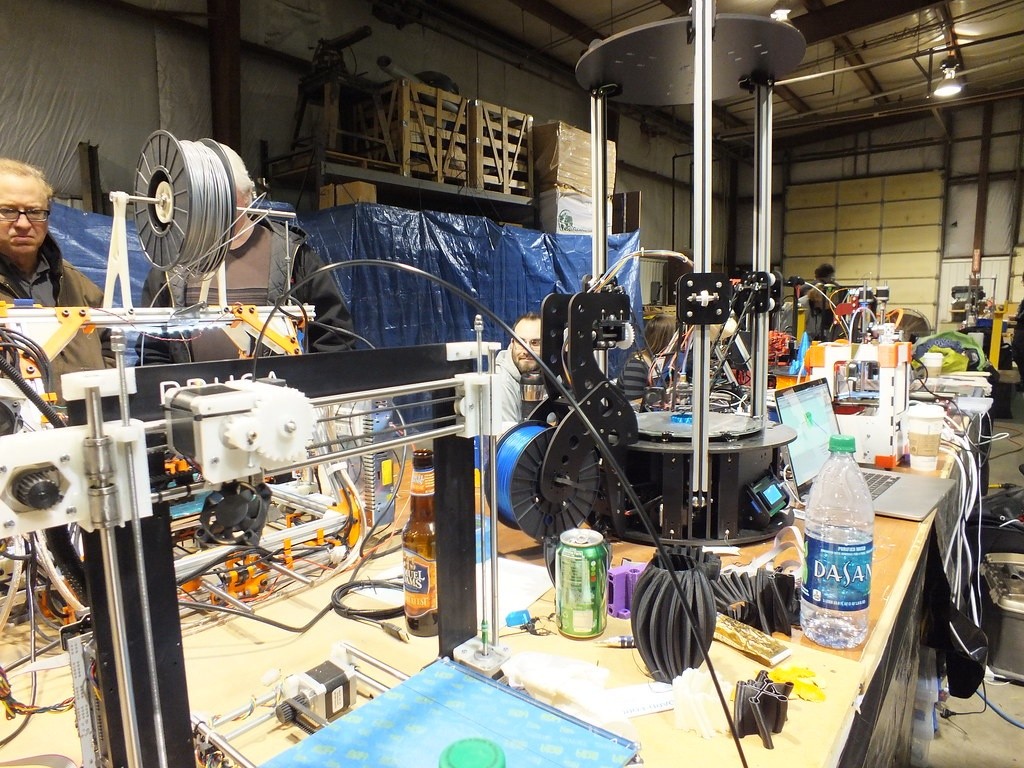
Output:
<box><xmin>359</xmin><ymin>78</ymin><xmax>536</xmax><ymax>198</ymax></box>
<box><xmin>979</xmin><ymin>553</ymin><xmax>1024</xmax><ymax>683</ymax></box>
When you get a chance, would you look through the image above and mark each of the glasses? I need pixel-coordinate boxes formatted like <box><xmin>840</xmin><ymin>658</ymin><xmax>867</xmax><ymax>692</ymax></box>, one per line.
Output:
<box><xmin>514</xmin><ymin>339</ymin><xmax>540</xmax><ymax>349</ymax></box>
<box><xmin>1</xmin><ymin>207</ymin><xmax>52</xmax><ymax>223</ymax></box>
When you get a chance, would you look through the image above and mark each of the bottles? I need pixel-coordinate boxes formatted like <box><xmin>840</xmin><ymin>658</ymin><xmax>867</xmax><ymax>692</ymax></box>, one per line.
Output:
<box><xmin>402</xmin><ymin>450</ymin><xmax>440</xmax><ymax>637</ymax></box>
<box><xmin>473</xmin><ymin>469</ymin><xmax>494</xmax><ymax>632</ymax></box>
<box><xmin>798</xmin><ymin>436</ymin><xmax>876</xmax><ymax>649</ymax></box>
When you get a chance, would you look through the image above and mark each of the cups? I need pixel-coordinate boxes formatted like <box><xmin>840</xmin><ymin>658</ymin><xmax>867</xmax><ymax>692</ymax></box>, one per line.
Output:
<box><xmin>905</xmin><ymin>405</ymin><xmax>944</xmax><ymax>470</ymax></box>
<box><xmin>922</xmin><ymin>353</ymin><xmax>945</xmax><ymax>378</ymax></box>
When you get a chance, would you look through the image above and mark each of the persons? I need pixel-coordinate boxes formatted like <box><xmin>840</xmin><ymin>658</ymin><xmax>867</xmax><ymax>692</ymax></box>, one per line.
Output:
<box><xmin>0</xmin><ymin>159</ymin><xmax>123</xmax><ymax>435</ymax></box>
<box><xmin>129</xmin><ymin>144</ymin><xmax>355</xmax><ymax>423</ymax></box>
<box><xmin>799</xmin><ymin>263</ymin><xmax>841</xmax><ymax>342</ymax></box>
<box><xmin>1009</xmin><ymin>272</ymin><xmax>1024</xmax><ymax>391</ymax></box>
<box><xmin>486</xmin><ymin>315</ymin><xmax>556</xmax><ymax>439</ymax></box>
<box><xmin>616</xmin><ymin>316</ymin><xmax>674</xmax><ymax>402</ymax></box>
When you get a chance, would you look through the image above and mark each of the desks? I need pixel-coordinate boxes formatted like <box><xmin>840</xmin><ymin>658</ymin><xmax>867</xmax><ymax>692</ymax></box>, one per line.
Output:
<box><xmin>482</xmin><ymin>407</ymin><xmax>969</xmax><ymax>766</ymax></box>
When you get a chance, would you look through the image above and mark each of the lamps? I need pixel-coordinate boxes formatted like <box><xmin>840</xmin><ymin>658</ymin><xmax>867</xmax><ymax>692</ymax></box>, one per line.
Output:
<box><xmin>933</xmin><ymin>57</ymin><xmax>962</xmax><ymax>98</ymax></box>
<box><xmin>770</xmin><ymin>5</ymin><xmax>791</xmax><ymax>21</ymax></box>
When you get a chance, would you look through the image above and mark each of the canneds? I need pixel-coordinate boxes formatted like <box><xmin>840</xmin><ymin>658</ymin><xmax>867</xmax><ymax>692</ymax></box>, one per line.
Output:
<box><xmin>555</xmin><ymin>528</ymin><xmax>607</xmax><ymax>639</ymax></box>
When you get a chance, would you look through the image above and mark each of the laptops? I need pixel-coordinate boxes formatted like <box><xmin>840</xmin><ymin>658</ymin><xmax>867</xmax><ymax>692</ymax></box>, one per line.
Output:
<box><xmin>775</xmin><ymin>377</ymin><xmax>957</xmax><ymax>521</ymax></box>
